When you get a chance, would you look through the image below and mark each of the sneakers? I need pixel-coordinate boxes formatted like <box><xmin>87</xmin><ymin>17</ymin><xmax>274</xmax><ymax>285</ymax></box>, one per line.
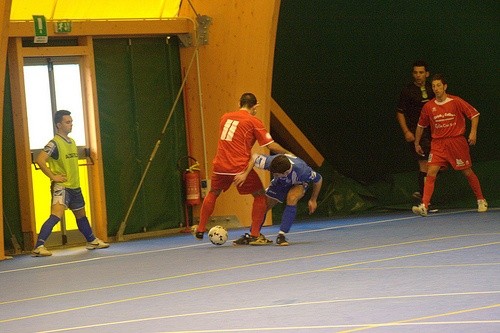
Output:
<box><xmin>412</xmin><ymin>191</ymin><xmax>422</xmax><ymax>202</ymax></box>
<box><xmin>248</xmin><ymin>233</ymin><xmax>274</xmax><ymax>244</ymax></box>
<box><xmin>233</xmin><ymin>233</ymin><xmax>252</xmax><ymax>245</ymax></box>
<box><xmin>276</xmin><ymin>230</ymin><xmax>289</xmax><ymax>246</ymax></box>
<box><xmin>30</xmin><ymin>245</ymin><xmax>53</xmax><ymax>257</ymax></box>
<box><xmin>191</xmin><ymin>225</ymin><xmax>204</xmax><ymax>239</ymax></box>
<box><xmin>477</xmin><ymin>199</ymin><xmax>488</xmax><ymax>212</ymax></box>
<box><xmin>86</xmin><ymin>238</ymin><xmax>109</xmax><ymax>250</ymax></box>
<box><xmin>412</xmin><ymin>203</ymin><xmax>428</xmax><ymax>216</ymax></box>
<box><xmin>427</xmin><ymin>203</ymin><xmax>439</xmax><ymax>213</ymax></box>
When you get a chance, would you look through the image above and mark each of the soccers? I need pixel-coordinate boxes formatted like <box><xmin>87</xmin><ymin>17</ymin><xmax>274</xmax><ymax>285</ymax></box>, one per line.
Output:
<box><xmin>207</xmin><ymin>224</ymin><xmax>229</xmax><ymax>246</ymax></box>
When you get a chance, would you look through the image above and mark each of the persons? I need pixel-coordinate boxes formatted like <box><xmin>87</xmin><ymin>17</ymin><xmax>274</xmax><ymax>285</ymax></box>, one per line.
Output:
<box><xmin>396</xmin><ymin>61</ymin><xmax>440</xmax><ymax>212</ymax></box>
<box><xmin>192</xmin><ymin>92</ymin><xmax>296</xmax><ymax>245</ymax></box>
<box><xmin>234</xmin><ymin>153</ymin><xmax>323</xmax><ymax>245</ymax></box>
<box><xmin>31</xmin><ymin>110</ymin><xmax>110</xmax><ymax>256</ymax></box>
<box><xmin>412</xmin><ymin>74</ymin><xmax>488</xmax><ymax>217</ymax></box>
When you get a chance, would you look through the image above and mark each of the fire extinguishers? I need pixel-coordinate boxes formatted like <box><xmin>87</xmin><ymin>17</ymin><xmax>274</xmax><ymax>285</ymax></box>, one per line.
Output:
<box><xmin>176</xmin><ymin>154</ymin><xmax>201</xmax><ymax>204</ymax></box>
